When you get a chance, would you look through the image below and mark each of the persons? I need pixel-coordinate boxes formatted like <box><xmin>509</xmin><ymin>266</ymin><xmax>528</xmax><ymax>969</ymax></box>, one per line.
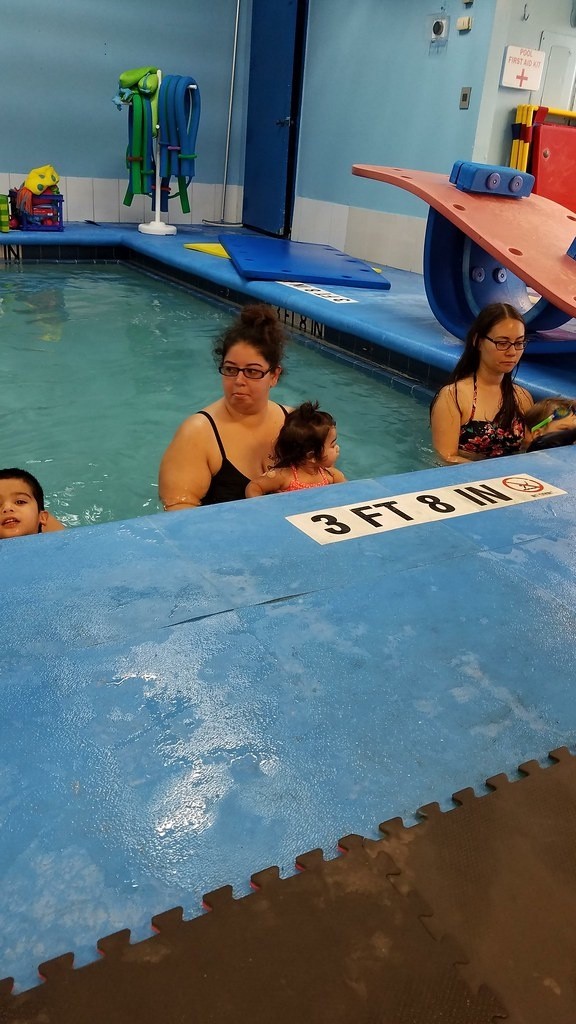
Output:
<box><xmin>429</xmin><ymin>303</ymin><xmax>576</xmax><ymax>463</ymax></box>
<box><xmin>0</xmin><ymin>468</ymin><xmax>65</xmax><ymax>540</ymax></box>
<box><xmin>158</xmin><ymin>301</ymin><xmax>297</xmax><ymax>513</ymax></box>
<box><xmin>244</xmin><ymin>399</ymin><xmax>349</xmax><ymax>499</ymax></box>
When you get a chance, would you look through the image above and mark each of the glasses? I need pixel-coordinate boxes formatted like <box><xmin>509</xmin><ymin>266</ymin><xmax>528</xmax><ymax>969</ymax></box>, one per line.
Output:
<box><xmin>219</xmin><ymin>365</ymin><xmax>275</xmax><ymax>379</ymax></box>
<box><xmin>483</xmin><ymin>334</ymin><xmax>529</xmax><ymax>351</ymax></box>
<box><xmin>531</xmin><ymin>407</ymin><xmax>570</xmax><ymax>431</ymax></box>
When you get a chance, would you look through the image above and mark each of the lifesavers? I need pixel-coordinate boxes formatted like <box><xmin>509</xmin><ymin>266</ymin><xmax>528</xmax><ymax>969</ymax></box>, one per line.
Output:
<box><xmin>110</xmin><ymin>66</ymin><xmax>201</xmax><ymax>214</ymax></box>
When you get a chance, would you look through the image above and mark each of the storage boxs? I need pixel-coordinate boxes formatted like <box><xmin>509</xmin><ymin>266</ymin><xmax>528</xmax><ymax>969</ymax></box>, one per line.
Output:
<box><xmin>8</xmin><ymin>189</ymin><xmax>65</xmax><ymax>232</ymax></box>
